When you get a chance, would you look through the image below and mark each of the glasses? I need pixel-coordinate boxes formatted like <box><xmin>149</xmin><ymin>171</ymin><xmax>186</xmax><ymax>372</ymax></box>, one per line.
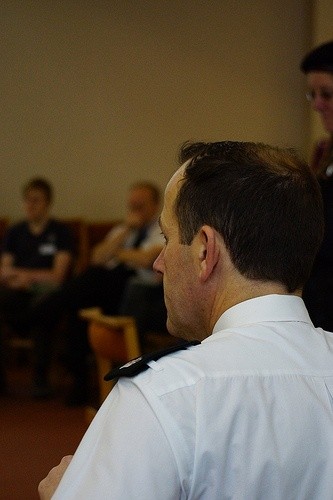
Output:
<box><xmin>305</xmin><ymin>88</ymin><xmax>333</xmax><ymax>102</ymax></box>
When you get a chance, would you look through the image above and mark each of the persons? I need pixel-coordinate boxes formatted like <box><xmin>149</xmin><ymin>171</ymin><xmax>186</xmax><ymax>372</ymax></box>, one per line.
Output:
<box><xmin>33</xmin><ymin>183</ymin><xmax>170</xmax><ymax>408</ymax></box>
<box><xmin>37</xmin><ymin>140</ymin><xmax>333</xmax><ymax>499</ymax></box>
<box><xmin>299</xmin><ymin>40</ymin><xmax>332</xmax><ymax>332</ymax></box>
<box><xmin>1</xmin><ymin>178</ymin><xmax>82</xmax><ymax>401</ymax></box>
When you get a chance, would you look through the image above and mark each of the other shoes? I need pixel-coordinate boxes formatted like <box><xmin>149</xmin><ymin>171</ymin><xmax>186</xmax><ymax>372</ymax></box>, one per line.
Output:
<box><xmin>68</xmin><ymin>382</ymin><xmax>85</xmax><ymax>403</ymax></box>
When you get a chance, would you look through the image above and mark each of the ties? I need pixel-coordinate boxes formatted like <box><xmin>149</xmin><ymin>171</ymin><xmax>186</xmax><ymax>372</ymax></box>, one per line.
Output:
<box><xmin>133</xmin><ymin>228</ymin><xmax>146</xmax><ymax>252</ymax></box>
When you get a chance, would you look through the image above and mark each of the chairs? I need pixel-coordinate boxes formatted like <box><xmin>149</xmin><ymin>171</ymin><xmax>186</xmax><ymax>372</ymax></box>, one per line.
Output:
<box><xmin>0</xmin><ymin>221</ymin><xmax>174</xmax><ymax>405</ymax></box>
